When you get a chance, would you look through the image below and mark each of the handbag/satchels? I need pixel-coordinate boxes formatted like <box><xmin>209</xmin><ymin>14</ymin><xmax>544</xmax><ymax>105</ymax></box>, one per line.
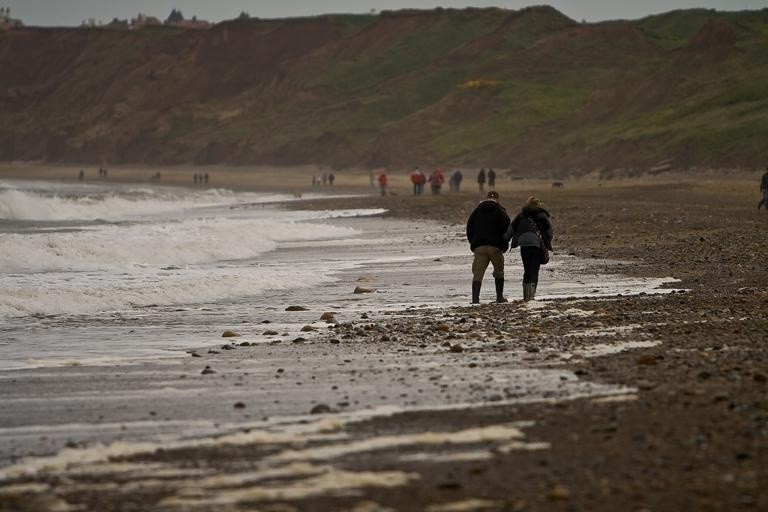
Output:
<box><xmin>538</xmin><ymin>247</ymin><xmax>550</xmax><ymax>265</ymax></box>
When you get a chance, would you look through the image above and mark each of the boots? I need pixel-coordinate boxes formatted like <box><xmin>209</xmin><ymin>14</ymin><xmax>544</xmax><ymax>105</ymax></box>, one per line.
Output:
<box><xmin>495</xmin><ymin>277</ymin><xmax>508</xmax><ymax>303</ymax></box>
<box><xmin>523</xmin><ymin>282</ymin><xmax>532</xmax><ymax>303</ymax></box>
<box><xmin>529</xmin><ymin>282</ymin><xmax>537</xmax><ymax>302</ymax></box>
<box><xmin>472</xmin><ymin>281</ymin><xmax>481</xmax><ymax>304</ymax></box>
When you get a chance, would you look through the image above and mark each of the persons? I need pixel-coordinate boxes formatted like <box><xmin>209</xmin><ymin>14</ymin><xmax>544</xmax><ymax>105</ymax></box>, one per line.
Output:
<box><xmin>757</xmin><ymin>167</ymin><xmax>767</xmax><ymax>210</ymax></box>
<box><xmin>78</xmin><ymin>170</ymin><xmax>85</xmax><ymax>181</ymax></box>
<box><xmin>465</xmin><ymin>190</ymin><xmax>513</xmax><ymax>304</ymax></box>
<box><xmin>204</xmin><ymin>173</ymin><xmax>208</xmax><ymax>183</ymax></box>
<box><xmin>411</xmin><ymin>167</ymin><xmax>497</xmax><ymax>195</ymax></box>
<box><xmin>104</xmin><ymin>169</ymin><xmax>108</xmax><ymax>176</ymax></box>
<box><xmin>503</xmin><ymin>195</ymin><xmax>553</xmax><ymax>302</ymax></box>
<box><xmin>198</xmin><ymin>173</ymin><xmax>204</xmax><ymax>184</ymax></box>
<box><xmin>193</xmin><ymin>173</ymin><xmax>198</xmax><ymax>183</ymax></box>
<box><xmin>156</xmin><ymin>171</ymin><xmax>160</xmax><ymax>178</ymax></box>
<box><xmin>99</xmin><ymin>168</ymin><xmax>102</xmax><ymax>176</ymax></box>
<box><xmin>312</xmin><ymin>174</ymin><xmax>335</xmax><ymax>187</ymax></box>
<box><xmin>368</xmin><ymin>169</ymin><xmax>374</xmax><ymax>187</ymax></box>
<box><xmin>376</xmin><ymin>172</ymin><xmax>387</xmax><ymax>195</ymax></box>
